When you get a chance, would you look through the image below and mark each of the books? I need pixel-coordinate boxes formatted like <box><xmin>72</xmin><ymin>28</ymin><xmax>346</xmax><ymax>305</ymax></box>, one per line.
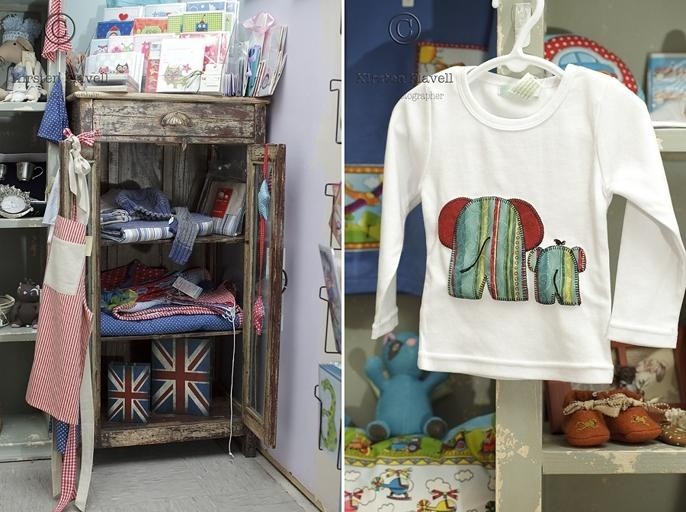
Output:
<box><xmin>82</xmin><ymin>0</ymin><xmax>289</xmax><ymax>99</ymax></box>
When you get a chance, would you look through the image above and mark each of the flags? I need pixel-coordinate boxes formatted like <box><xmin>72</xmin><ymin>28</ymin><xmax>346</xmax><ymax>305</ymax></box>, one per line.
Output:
<box><xmin>0</xmin><ymin>13</ymin><xmax>48</xmax><ymax>102</ymax></box>
<box><xmin>8</xmin><ymin>278</ymin><xmax>40</xmax><ymax>329</ymax></box>
<box><xmin>363</xmin><ymin>330</ymin><xmax>453</xmax><ymax>444</ymax></box>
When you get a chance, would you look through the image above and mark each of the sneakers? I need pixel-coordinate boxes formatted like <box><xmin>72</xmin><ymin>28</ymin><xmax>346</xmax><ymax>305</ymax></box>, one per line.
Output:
<box><xmin>607</xmin><ymin>388</ymin><xmax>663</xmax><ymax>443</ymax></box>
<box><xmin>645</xmin><ymin>400</ymin><xmax>686</xmax><ymax>446</ymax></box>
<box><xmin>563</xmin><ymin>387</ymin><xmax>612</xmax><ymax>448</ymax></box>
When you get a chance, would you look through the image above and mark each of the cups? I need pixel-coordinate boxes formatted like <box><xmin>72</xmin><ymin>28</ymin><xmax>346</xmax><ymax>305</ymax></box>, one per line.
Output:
<box><xmin>14</xmin><ymin>161</ymin><xmax>44</xmax><ymax>182</ymax></box>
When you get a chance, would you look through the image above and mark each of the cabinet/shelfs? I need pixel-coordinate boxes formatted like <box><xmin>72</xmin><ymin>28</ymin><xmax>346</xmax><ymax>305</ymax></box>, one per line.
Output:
<box><xmin>0</xmin><ymin>0</ymin><xmax>290</xmax><ymax>468</ymax></box>
<box><xmin>344</xmin><ymin>1</ymin><xmax>686</xmax><ymax>512</ymax></box>
